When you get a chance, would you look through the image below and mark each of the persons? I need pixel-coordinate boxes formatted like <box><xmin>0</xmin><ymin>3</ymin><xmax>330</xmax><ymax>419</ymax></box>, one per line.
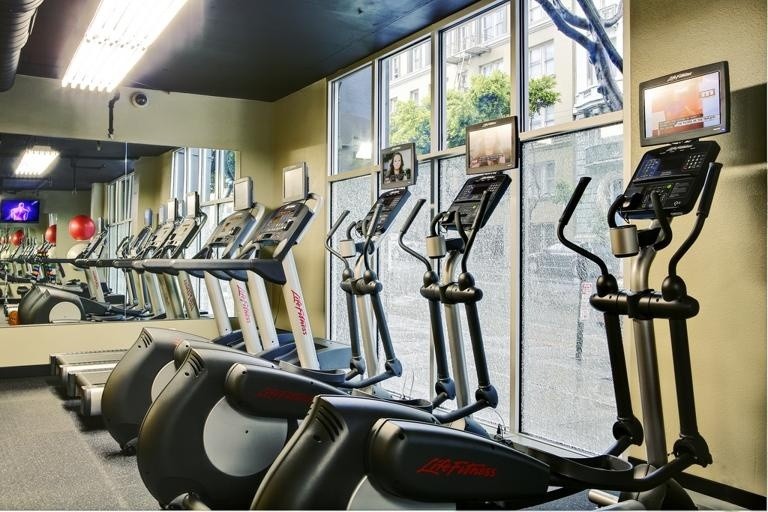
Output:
<box><xmin>11</xmin><ymin>202</ymin><xmax>29</xmax><ymax>222</ymax></box>
<box><xmin>470</xmin><ymin>128</ymin><xmax>505</xmax><ymax>166</ymax></box>
<box><xmin>384</xmin><ymin>152</ymin><xmax>407</xmax><ymax>184</ymax></box>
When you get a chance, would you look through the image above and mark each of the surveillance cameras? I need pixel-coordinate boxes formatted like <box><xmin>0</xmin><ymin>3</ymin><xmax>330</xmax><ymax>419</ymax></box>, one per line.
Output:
<box><xmin>131</xmin><ymin>92</ymin><xmax>150</xmax><ymax>108</ymax></box>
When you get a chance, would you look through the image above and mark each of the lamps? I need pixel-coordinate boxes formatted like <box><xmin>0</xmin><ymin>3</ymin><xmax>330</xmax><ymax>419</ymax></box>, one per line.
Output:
<box><xmin>12</xmin><ymin>149</ymin><xmax>61</xmax><ymax>178</ymax></box>
<box><xmin>62</xmin><ymin>0</ymin><xmax>189</xmax><ymax>94</ymax></box>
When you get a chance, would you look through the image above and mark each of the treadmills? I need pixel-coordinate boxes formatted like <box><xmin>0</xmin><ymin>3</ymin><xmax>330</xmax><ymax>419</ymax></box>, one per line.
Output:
<box><xmin>50</xmin><ymin>176</ymin><xmax>274</xmax><ymax>389</ymax></box>
<box><xmin>67</xmin><ymin>164</ymin><xmax>354</xmax><ymax>417</ymax></box>
<box><xmin>50</xmin><ymin>192</ymin><xmax>208</xmax><ymax>326</ymax></box>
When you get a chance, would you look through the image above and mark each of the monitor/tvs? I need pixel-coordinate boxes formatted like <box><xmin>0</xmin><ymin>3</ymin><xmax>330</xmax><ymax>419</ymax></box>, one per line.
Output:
<box><xmin>167</xmin><ymin>197</ymin><xmax>178</xmax><ymax>222</ymax></box>
<box><xmin>466</xmin><ymin>116</ymin><xmax>518</xmax><ymax>175</ymax></box>
<box><xmin>184</xmin><ymin>191</ymin><xmax>200</xmax><ymax>217</ymax></box>
<box><xmin>0</xmin><ymin>199</ymin><xmax>40</xmax><ymax>223</ymax></box>
<box><xmin>282</xmin><ymin>161</ymin><xmax>310</xmax><ymax>202</ymax></box>
<box><xmin>98</xmin><ymin>217</ymin><xmax>104</xmax><ymax>233</ymax></box>
<box><xmin>636</xmin><ymin>60</ymin><xmax>731</xmax><ymax>147</ymax></box>
<box><xmin>143</xmin><ymin>207</ymin><xmax>153</xmax><ymax>227</ymax></box>
<box><xmin>158</xmin><ymin>204</ymin><xmax>166</xmax><ymax>225</ymax></box>
<box><xmin>380</xmin><ymin>142</ymin><xmax>418</xmax><ymax>190</ymax></box>
<box><xmin>232</xmin><ymin>176</ymin><xmax>253</xmax><ymax>211</ymax></box>
